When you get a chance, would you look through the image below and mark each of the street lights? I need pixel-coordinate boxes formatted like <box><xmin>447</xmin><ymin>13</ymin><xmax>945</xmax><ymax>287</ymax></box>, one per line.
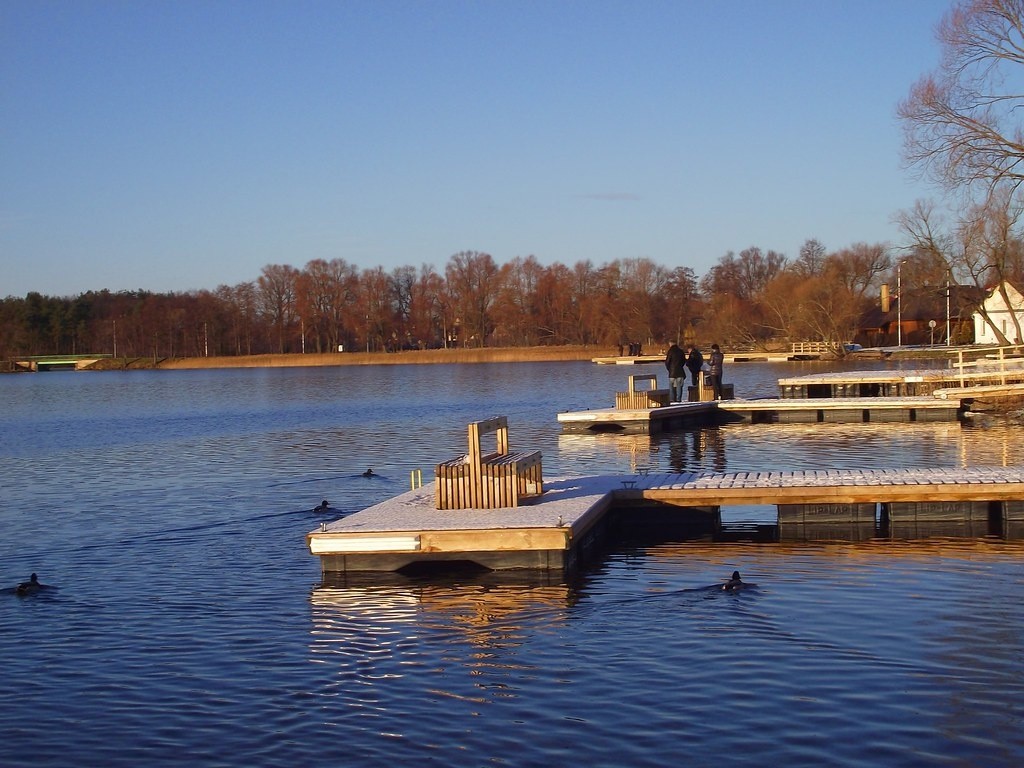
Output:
<box><xmin>897</xmin><ymin>260</ymin><xmax>907</xmax><ymax>347</ymax></box>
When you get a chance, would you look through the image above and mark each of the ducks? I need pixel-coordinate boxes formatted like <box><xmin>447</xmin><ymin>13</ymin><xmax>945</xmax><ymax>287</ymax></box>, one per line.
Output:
<box><xmin>313</xmin><ymin>500</ymin><xmax>330</xmax><ymax>511</ymax></box>
<box><xmin>17</xmin><ymin>572</ymin><xmax>41</xmax><ymax>591</ymax></box>
<box><xmin>363</xmin><ymin>468</ymin><xmax>373</xmax><ymax>476</ymax></box>
<box><xmin>722</xmin><ymin>570</ymin><xmax>743</xmax><ymax>590</ymax></box>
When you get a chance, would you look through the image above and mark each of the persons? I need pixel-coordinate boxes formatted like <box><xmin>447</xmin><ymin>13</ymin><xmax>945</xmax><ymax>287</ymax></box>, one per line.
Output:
<box><xmin>664</xmin><ymin>339</ymin><xmax>686</xmax><ymax>403</ymax></box>
<box><xmin>618</xmin><ymin>345</ymin><xmax>624</xmax><ymax>357</ymax></box>
<box><xmin>706</xmin><ymin>344</ymin><xmax>724</xmax><ymax>401</ymax></box>
<box><xmin>628</xmin><ymin>341</ymin><xmax>641</xmax><ymax>357</ymax></box>
<box><xmin>684</xmin><ymin>344</ymin><xmax>704</xmax><ymax>386</ymax></box>
